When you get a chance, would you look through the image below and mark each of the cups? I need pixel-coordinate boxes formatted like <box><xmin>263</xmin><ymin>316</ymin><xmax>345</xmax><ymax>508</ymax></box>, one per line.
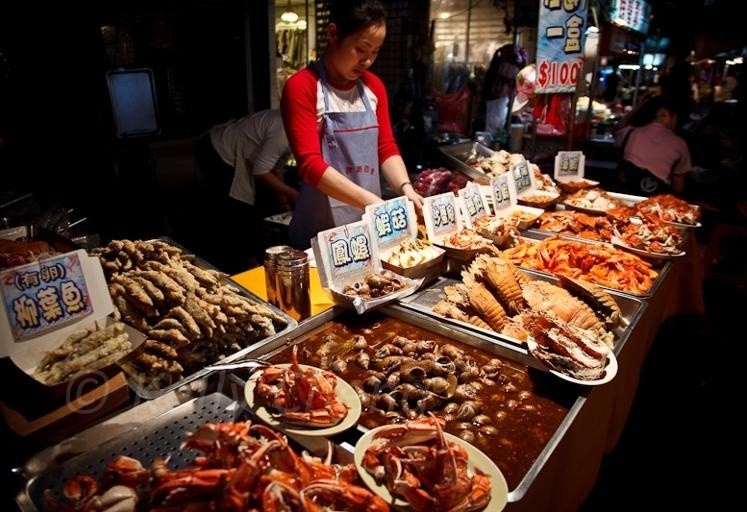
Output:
<box><xmin>509</xmin><ymin>123</ymin><xmax>525</xmax><ymax>153</ymax></box>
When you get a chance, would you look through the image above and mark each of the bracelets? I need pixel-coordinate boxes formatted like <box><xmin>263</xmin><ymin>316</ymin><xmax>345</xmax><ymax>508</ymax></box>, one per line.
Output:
<box><xmin>400</xmin><ymin>182</ymin><xmax>411</xmax><ymax>194</ymax></box>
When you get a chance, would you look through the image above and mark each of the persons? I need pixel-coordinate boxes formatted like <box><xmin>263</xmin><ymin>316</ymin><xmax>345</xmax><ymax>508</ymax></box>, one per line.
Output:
<box><xmin>194</xmin><ymin>107</ymin><xmax>300</xmax><ymax>212</ymax></box>
<box><xmin>278</xmin><ymin>0</ymin><xmax>425</xmax><ymax>253</ymax></box>
<box><xmin>390</xmin><ymin>88</ymin><xmax>425</xmax><ymax>174</ymax></box>
<box><xmin>595</xmin><ymin>62</ymin><xmax>692</xmax><ymax>199</ymax></box>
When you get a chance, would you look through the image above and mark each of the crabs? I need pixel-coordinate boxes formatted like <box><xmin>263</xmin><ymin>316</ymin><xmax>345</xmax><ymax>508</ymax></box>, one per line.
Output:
<box><xmin>33</xmin><ymin>344</ymin><xmax>495</xmax><ymax>511</ymax></box>
<box><xmin>506</xmin><ymin>184</ymin><xmax>702</xmax><ymax>295</ymax></box>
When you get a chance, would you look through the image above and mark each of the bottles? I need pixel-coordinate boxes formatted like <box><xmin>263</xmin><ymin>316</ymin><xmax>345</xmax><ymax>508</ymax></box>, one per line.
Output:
<box><xmin>590</xmin><ymin>117</ymin><xmax>613</xmax><ymax>140</ymax></box>
<box><xmin>262</xmin><ymin>243</ymin><xmax>311</xmax><ymax>324</ymax></box>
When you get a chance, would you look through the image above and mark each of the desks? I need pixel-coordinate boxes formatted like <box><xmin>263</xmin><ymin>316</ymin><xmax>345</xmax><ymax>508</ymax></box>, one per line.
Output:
<box><xmin>231</xmin><ymin>265</ymin><xmax>335</xmax><ymax>325</ymax></box>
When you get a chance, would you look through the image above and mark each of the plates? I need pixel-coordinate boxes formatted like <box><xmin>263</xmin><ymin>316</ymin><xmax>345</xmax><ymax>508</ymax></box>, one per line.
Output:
<box><xmin>351</xmin><ymin>422</ymin><xmax>511</xmax><ymax>511</ymax></box>
<box><xmin>561</xmin><ymin>201</ymin><xmax>609</xmax><ymax>215</ymax></box>
<box><xmin>611</xmin><ymin>236</ymin><xmax>686</xmax><ymax>258</ymax></box>
<box><xmin>639</xmin><ymin>213</ymin><xmax>703</xmax><ymax>229</ymax></box>
<box><xmin>241</xmin><ymin>362</ymin><xmax>364</xmax><ymax>437</ymax></box>
